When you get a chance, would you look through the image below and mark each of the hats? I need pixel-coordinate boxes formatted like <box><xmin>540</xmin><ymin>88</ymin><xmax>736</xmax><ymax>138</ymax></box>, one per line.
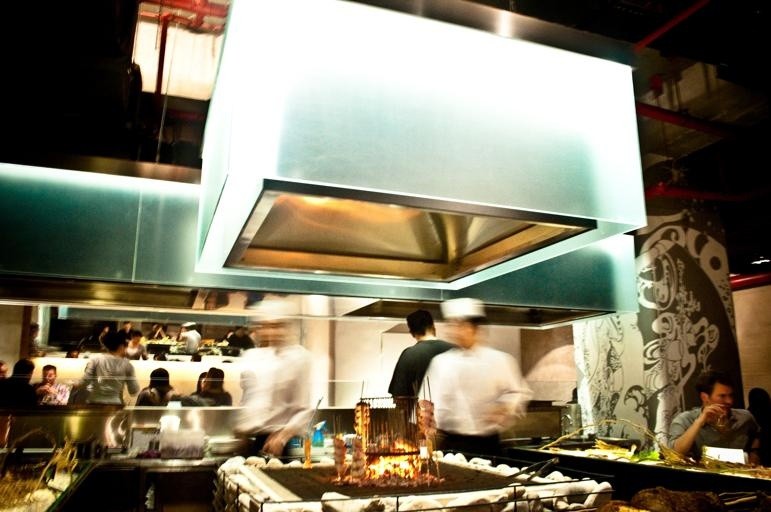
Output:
<box><xmin>245</xmin><ymin>298</ymin><xmax>301</xmax><ymax>322</ymax></box>
<box><xmin>181</xmin><ymin>321</ymin><xmax>197</xmax><ymax>327</ymax></box>
<box><xmin>440</xmin><ymin>298</ymin><xmax>488</xmax><ymax>319</ymax></box>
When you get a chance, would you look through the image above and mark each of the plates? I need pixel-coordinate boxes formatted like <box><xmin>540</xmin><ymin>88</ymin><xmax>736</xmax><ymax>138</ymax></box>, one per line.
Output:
<box><xmin>209</xmin><ymin>438</ymin><xmax>246</xmax><ymax>453</ymax></box>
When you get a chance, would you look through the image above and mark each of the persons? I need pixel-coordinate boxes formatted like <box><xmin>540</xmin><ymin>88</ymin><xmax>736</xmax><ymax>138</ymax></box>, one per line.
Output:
<box><xmin>23</xmin><ymin>319</ymin><xmax>255</xmax><ymax>362</ymax></box>
<box><xmin>388</xmin><ymin>310</ymin><xmax>463</xmax><ymax>409</ymax></box>
<box><xmin>0</xmin><ymin>331</ymin><xmax>233</xmax><ymax>411</ymax></box>
<box><xmin>668</xmin><ymin>371</ymin><xmax>763</xmax><ymax>465</ymax></box>
<box><xmin>233</xmin><ymin>300</ymin><xmax>317</xmax><ymax>466</ymax></box>
<box><xmin>419</xmin><ymin>297</ymin><xmax>534</xmax><ymax>464</ymax></box>
<box><xmin>747</xmin><ymin>387</ymin><xmax>771</xmax><ymax>429</ymax></box>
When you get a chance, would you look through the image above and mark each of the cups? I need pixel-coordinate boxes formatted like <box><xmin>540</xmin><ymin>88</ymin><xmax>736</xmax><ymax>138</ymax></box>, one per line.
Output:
<box><xmin>715</xmin><ymin>405</ymin><xmax>729</xmax><ymax>429</ymax></box>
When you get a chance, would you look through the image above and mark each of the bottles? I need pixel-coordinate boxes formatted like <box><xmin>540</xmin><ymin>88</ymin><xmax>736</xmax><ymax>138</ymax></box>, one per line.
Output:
<box><xmin>148</xmin><ymin>439</ymin><xmax>159</xmax><ymax>450</ymax></box>
<box><xmin>312</xmin><ymin>420</ymin><xmax>327</xmax><ymax>447</ymax></box>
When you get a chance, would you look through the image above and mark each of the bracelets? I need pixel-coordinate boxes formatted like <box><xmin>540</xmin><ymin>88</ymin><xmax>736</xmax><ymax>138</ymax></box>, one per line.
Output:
<box><xmin>749</xmin><ymin>448</ymin><xmax>759</xmax><ymax>453</ymax></box>
